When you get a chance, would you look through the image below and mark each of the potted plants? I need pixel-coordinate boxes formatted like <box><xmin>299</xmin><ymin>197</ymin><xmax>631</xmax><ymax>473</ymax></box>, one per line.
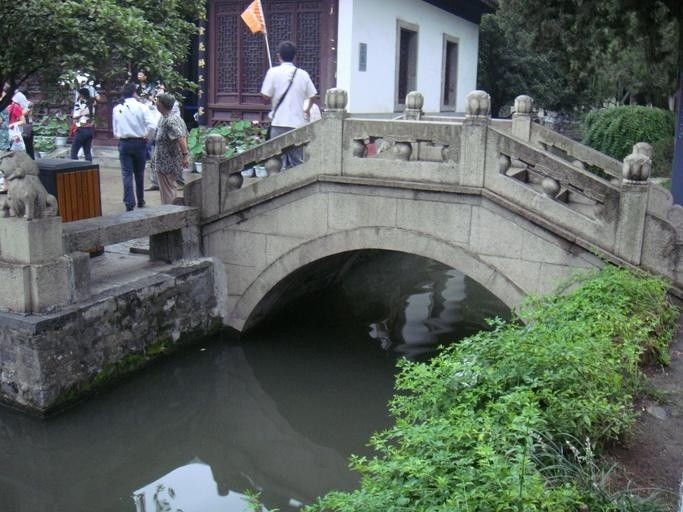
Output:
<box><xmin>187</xmin><ymin>120</ymin><xmax>270</xmax><ymax>177</ymax></box>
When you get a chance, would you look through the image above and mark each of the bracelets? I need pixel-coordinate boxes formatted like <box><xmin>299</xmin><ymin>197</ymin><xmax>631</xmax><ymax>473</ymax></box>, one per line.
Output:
<box><xmin>181</xmin><ymin>151</ymin><xmax>187</xmax><ymax>156</ymax></box>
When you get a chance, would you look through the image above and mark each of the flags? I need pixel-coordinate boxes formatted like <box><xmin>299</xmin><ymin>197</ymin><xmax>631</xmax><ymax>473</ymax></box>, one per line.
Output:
<box><xmin>240</xmin><ymin>0</ymin><xmax>264</xmax><ymax>34</ymax></box>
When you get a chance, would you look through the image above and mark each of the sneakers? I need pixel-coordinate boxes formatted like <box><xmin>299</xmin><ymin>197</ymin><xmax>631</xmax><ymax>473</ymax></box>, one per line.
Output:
<box><xmin>123</xmin><ymin>199</ymin><xmax>146</xmax><ymax>213</ymax></box>
<box><xmin>141</xmin><ymin>182</ymin><xmax>159</xmax><ymax>192</ymax></box>
<box><xmin>175</xmin><ymin>182</ymin><xmax>185</xmax><ymax>192</ymax></box>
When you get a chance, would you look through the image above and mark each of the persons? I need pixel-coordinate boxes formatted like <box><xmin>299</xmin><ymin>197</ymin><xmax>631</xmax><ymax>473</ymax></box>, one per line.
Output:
<box><xmin>111</xmin><ymin>83</ymin><xmax>155</xmax><ymax>211</ymax></box>
<box><xmin>152</xmin><ymin>92</ymin><xmax>189</xmax><ymax>204</ymax></box>
<box><xmin>69</xmin><ymin>88</ymin><xmax>93</xmax><ymax>162</ymax></box>
<box><xmin>8</xmin><ymin>99</ymin><xmax>27</xmax><ymax>153</ymax></box>
<box><xmin>259</xmin><ymin>41</ymin><xmax>317</xmax><ymax>171</ymax></box>
<box><xmin>131</xmin><ymin>69</ymin><xmax>154</xmax><ymax>101</ymax></box>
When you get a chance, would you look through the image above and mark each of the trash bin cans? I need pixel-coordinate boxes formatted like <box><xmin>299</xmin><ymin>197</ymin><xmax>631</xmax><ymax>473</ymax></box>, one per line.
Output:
<box><xmin>32</xmin><ymin>158</ymin><xmax>104</xmax><ymax>258</ymax></box>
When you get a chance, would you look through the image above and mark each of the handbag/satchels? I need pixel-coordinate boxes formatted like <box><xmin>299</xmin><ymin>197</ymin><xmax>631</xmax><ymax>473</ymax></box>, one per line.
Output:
<box><xmin>263</xmin><ymin>124</ymin><xmax>272</xmax><ymax>142</ymax></box>
